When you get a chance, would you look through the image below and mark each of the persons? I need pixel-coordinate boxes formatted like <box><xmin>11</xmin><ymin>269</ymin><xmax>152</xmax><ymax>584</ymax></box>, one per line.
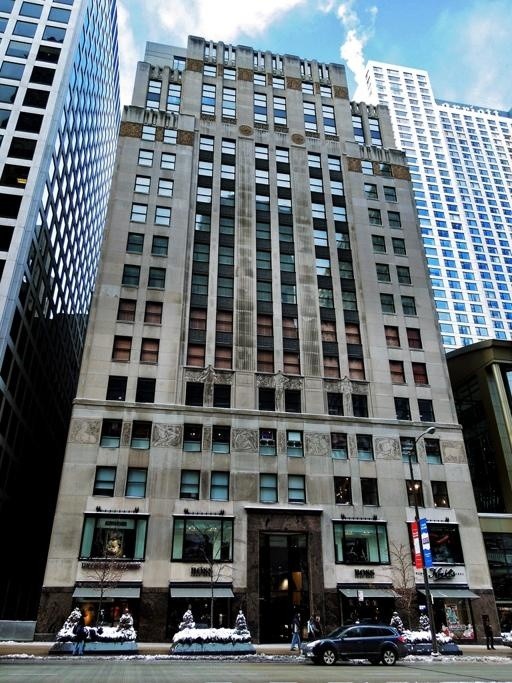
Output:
<box><xmin>307</xmin><ymin>616</ymin><xmax>319</xmax><ymax>640</ymax></box>
<box><xmin>71</xmin><ymin>618</ymin><xmax>85</xmax><ymax>656</ymax></box>
<box><xmin>485</xmin><ymin>624</ymin><xmax>495</xmax><ymax>650</ymax></box>
<box><xmin>291</xmin><ymin>612</ymin><xmax>302</xmax><ymax>651</ymax></box>
<box><xmin>314</xmin><ymin>615</ymin><xmax>322</xmax><ymax>639</ymax></box>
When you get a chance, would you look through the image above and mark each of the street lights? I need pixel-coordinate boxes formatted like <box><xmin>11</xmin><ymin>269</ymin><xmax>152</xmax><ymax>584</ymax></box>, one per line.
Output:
<box><xmin>408</xmin><ymin>427</ymin><xmax>439</xmax><ymax>657</ymax></box>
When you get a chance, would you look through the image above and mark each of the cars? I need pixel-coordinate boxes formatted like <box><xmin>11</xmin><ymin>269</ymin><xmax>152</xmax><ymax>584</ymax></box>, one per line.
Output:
<box><xmin>301</xmin><ymin>623</ymin><xmax>410</xmax><ymax>665</ymax></box>
